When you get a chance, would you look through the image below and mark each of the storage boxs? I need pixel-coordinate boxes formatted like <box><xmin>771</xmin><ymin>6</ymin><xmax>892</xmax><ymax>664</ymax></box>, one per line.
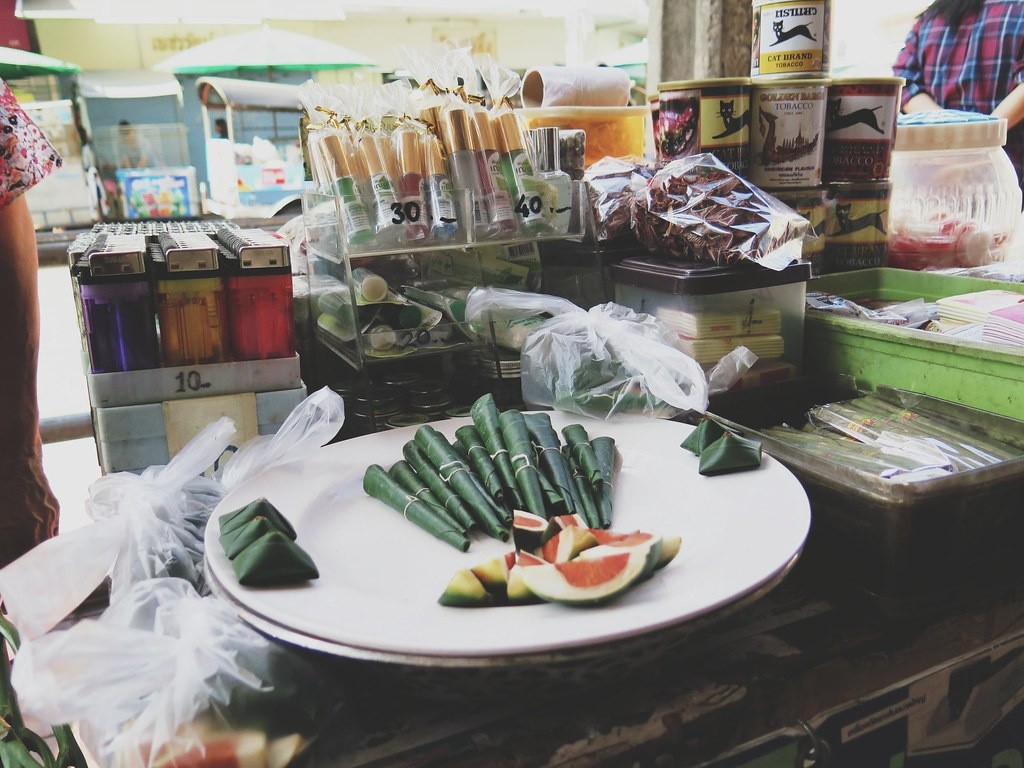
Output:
<box><xmin>606</xmin><ymin>250</ymin><xmax>811</xmax><ymax>426</ymax></box>
<box><xmin>801</xmin><ymin>265</ymin><xmax>1024</xmax><ymax>418</ymax></box>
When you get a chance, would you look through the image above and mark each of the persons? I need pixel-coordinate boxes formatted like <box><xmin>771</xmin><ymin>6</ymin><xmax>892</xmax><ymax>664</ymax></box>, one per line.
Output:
<box><xmin>0</xmin><ymin>79</ymin><xmax>63</xmax><ymax>573</ymax></box>
<box><xmin>889</xmin><ymin>0</ymin><xmax>1024</xmax><ymax>197</ymax></box>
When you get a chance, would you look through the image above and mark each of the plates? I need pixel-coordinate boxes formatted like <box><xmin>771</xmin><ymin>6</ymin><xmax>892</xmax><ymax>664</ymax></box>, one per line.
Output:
<box><xmin>201</xmin><ymin>410</ymin><xmax>811</xmax><ymax>669</ymax></box>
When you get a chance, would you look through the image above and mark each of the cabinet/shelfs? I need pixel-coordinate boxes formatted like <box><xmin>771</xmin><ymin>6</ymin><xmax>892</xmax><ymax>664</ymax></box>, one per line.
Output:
<box><xmin>303</xmin><ymin>189</ymin><xmax>604</xmax><ymax>447</ymax></box>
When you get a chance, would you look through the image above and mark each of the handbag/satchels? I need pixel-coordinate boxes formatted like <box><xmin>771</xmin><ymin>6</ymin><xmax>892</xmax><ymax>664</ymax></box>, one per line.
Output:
<box><xmin>465</xmin><ymin>285</ymin><xmax>759</xmax><ymax>420</ymax></box>
<box><xmin>0</xmin><ymin>384</ymin><xmax>347</xmax><ymax>768</ymax></box>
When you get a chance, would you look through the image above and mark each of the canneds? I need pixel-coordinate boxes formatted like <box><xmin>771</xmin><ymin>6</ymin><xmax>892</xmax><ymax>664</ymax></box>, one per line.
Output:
<box><xmin>657</xmin><ymin>0</ymin><xmax>906</xmax><ymax>275</ymax></box>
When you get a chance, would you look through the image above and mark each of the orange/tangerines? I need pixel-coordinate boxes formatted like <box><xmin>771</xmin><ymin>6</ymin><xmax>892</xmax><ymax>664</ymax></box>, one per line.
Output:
<box><xmin>506</xmin><ymin>508</ymin><xmax>682</xmax><ymax>603</ymax></box>
